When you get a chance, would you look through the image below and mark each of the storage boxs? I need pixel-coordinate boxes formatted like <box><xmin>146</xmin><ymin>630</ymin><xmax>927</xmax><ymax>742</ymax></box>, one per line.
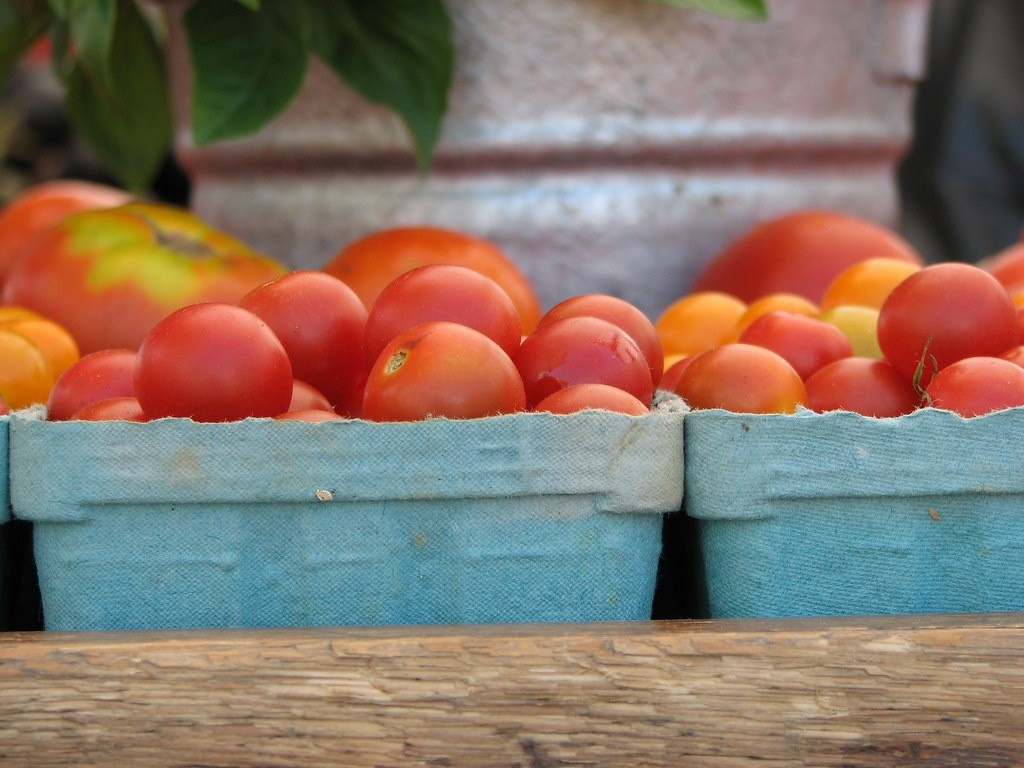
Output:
<box><xmin>0</xmin><ymin>384</ymin><xmax>1024</xmax><ymax>636</ymax></box>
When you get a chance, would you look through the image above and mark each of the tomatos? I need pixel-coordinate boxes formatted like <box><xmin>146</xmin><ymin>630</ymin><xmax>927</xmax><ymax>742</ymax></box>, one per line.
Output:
<box><xmin>45</xmin><ymin>260</ymin><xmax>1024</xmax><ymax>422</ymax></box>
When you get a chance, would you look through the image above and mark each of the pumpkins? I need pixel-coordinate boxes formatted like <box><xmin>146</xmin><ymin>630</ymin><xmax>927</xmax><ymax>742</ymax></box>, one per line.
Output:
<box><xmin>0</xmin><ymin>182</ymin><xmax>924</xmax><ymax>414</ymax></box>
